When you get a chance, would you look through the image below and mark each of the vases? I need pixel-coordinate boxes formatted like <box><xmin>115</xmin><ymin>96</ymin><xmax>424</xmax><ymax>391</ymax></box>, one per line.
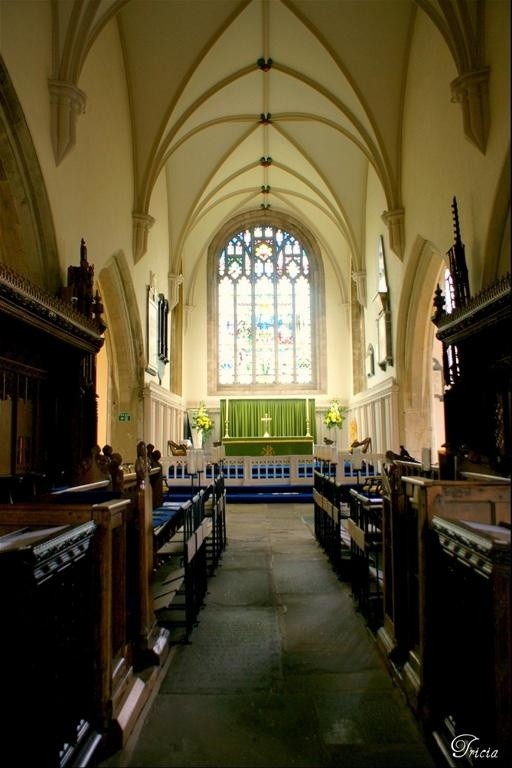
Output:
<box><xmin>330</xmin><ymin>429</ymin><xmax>341</xmax><ymax>448</ymax></box>
<box><xmin>191</xmin><ymin>428</ymin><xmax>203</xmax><ymax>449</ymax></box>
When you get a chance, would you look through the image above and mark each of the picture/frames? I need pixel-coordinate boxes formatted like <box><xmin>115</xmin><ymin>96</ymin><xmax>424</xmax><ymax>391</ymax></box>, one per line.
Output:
<box><xmin>143</xmin><ymin>286</ymin><xmax>170</xmax><ymax>379</ymax></box>
<box><xmin>363</xmin><ymin>310</ymin><xmax>396</xmax><ymax>381</ymax></box>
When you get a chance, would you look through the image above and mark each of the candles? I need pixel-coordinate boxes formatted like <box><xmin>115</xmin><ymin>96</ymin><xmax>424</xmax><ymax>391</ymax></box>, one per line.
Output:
<box><xmin>306</xmin><ymin>398</ymin><xmax>309</xmax><ymax>419</ymax></box>
<box><xmin>225</xmin><ymin>398</ymin><xmax>230</xmax><ymax>421</ymax></box>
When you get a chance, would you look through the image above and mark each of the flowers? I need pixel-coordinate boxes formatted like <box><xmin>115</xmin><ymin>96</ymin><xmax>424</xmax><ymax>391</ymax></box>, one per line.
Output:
<box><xmin>191</xmin><ymin>399</ymin><xmax>215</xmax><ymax>435</ymax></box>
<box><xmin>323</xmin><ymin>397</ymin><xmax>352</xmax><ymax>429</ymax></box>
